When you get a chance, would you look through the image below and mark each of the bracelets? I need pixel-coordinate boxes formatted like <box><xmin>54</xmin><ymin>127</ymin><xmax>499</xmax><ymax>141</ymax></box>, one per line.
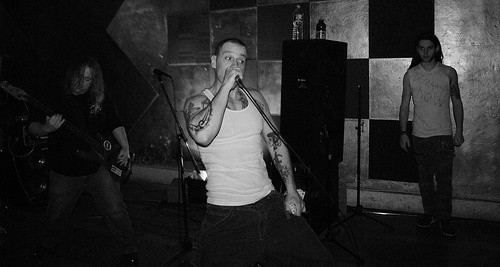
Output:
<box><xmin>400</xmin><ymin>131</ymin><xmax>406</xmax><ymax>133</ymax></box>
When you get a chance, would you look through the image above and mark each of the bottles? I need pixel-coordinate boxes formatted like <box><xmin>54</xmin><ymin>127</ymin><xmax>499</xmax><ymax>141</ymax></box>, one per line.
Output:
<box><xmin>315</xmin><ymin>19</ymin><xmax>327</xmax><ymax>39</ymax></box>
<box><xmin>292</xmin><ymin>5</ymin><xmax>304</xmax><ymax>40</ymax></box>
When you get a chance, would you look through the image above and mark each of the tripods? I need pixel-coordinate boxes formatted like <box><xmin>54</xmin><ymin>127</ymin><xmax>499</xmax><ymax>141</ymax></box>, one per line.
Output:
<box><xmin>236</xmin><ymin>57</ymin><xmax>396</xmax><ymax>265</ymax></box>
<box><xmin>157</xmin><ymin>74</ymin><xmax>198</xmax><ymax>267</ymax></box>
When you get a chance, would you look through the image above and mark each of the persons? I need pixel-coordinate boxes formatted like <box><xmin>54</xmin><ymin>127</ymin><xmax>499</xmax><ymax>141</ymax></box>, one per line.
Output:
<box><xmin>26</xmin><ymin>55</ymin><xmax>148</xmax><ymax>267</ymax></box>
<box><xmin>184</xmin><ymin>38</ymin><xmax>307</xmax><ymax>267</ymax></box>
<box><xmin>400</xmin><ymin>34</ymin><xmax>465</xmax><ymax>236</ymax></box>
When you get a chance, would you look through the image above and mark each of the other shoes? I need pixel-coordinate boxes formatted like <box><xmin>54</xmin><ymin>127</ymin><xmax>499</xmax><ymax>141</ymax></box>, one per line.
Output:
<box><xmin>439</xmin><ymin>223</ymin><xmax>456</xmax><ymax>240</ymax></box>
<box><xmin>416</xmin><ymin>213</ymin><xmax>437</xmax><ymax>228</ymax></box>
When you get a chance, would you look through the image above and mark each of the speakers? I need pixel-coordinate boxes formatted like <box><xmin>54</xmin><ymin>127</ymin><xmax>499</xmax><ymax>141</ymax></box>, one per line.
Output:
<box><xmin>268</xmin><ymin>162</ymin><xmax>339</xmax><ymax>235</ymax></box>
<box><xmin>277</xmin><ymin>39</ymin><xmax>348</xmax><ymax>166</ymax></box>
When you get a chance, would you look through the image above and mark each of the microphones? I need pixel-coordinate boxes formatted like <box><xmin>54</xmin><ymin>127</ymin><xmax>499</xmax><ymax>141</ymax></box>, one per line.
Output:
<box><xmin>149</xmin><ymin>66</ymin><xmax>172</xmax><ymax>77</ymax></box>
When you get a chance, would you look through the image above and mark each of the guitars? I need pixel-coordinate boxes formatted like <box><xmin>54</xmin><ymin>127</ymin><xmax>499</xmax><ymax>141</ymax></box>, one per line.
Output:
<box><xmin>0</xmin><ymin>79</ymin><xmax>132</xmax><ymax>183</ymax></box>
